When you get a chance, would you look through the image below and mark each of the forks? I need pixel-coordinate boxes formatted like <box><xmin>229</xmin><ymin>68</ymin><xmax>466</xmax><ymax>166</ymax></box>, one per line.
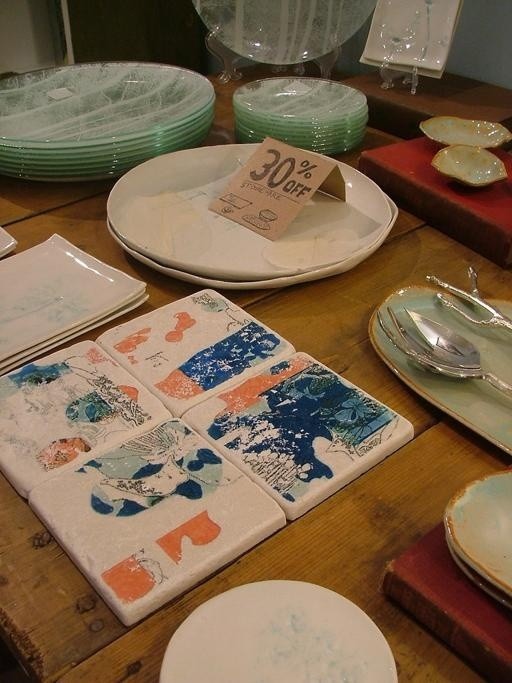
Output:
<box><xmin>374</xmin><ymin>296</ymin><xmax>512</xmax><ymax>404</ymax></box>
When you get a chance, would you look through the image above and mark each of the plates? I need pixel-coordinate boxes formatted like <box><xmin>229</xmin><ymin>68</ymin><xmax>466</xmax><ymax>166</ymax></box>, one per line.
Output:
<box><xmin>231</xmin><ymin>76</ymin><xmax>371</xmax><ymax>157</ymax></box>
<box><xmin>429</xmin><ymin>145</ymin><xmax>509</xmax><ymax>187</ymax></box>
<box><xmin>419</xmin><ymin>116</ymin><xmax>511</xmax><ymax>147</ymax></box>
<box><xmin>365</xmin><ymin>272</ymin><xmax>512</xmax><ymax>455</ymax></box>
<box><xmin>192</xmin><ymin>1</ymin><xmax>378</xmax><ymax>84</ymax></box>
<box><xmin>441</xmin><ymin>470</ymin><xmax>512</xmax><ymax>610</ymax></box>
<box><xmin>1</xmin><ymin>228</ymin><xmax>154</xmax><ymax>377</ymax></box>
<box><xmin>107</xmin><ymin>141</ymin><xmax>400</xmax><ymax>290</ymax></box>
<box><xmin>0</xmin><ymin>61</ymin><xmax>217</xmax><ymax>183</ymax></box>
